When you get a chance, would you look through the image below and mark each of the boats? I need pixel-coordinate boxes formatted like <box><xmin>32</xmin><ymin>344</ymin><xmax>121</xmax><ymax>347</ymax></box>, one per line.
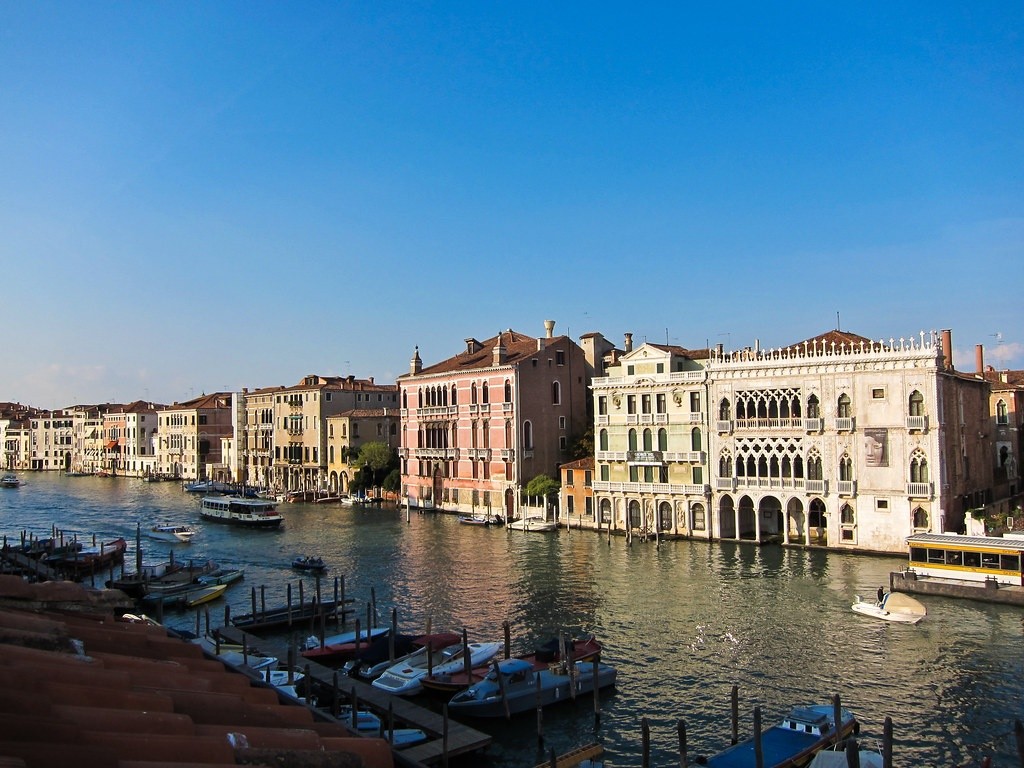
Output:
<box><xmin>148</xmin><ymin>523</ymin><xmax>192</xmax><ymax>543</ymax></box>
<box><xmin>686</xmin><ymin>705</ymin><xmax>860</xmax><ymax>768</ymax></box>
<box><xmin>338</xmin><ymin>705</ymin><xmax>380</xmax><ymax>738</ymax></box>
<box><xmin>383</xmin><ymin>730</ymin><xmax>427</xmax><ymax>751</ymax></box>
<box><xmin>297</xmin><ymin>625</ymin><xmax>390</xmax><ymax>652</ymax></box>
<box><xmin>508</xmin><ymin>515</ymin><xmax>560</xmax><ymax>531</ymax></box>
<box><xmin>105</xmin><ymin>556</ymin><xmax>245</xmax><ymax>605</ymax></box>
<box><xmin>184</xmin><ymin>479</ymin><xmax>255</xmax><ymax>494</ymax></box>
<box><xmin>330</xmin><ymin>632</ymin><xmax>461</xmax><ymax>684</ymax></box>
<box><xmin>418</xmin><ymin>630</ymin><xmax>603</xmax><ymax>708</ymax></box>
<box><xmin>193</xmin><ymin>630</ymin><xmax>320</xmax><ymax>705</ymax></box>
<box><xmin>371</xmin><ymin>639</ymin><xmax>505</xmax><ymax>703</ymax></box>
<box><xmin>255</xmin><ymin>489</ymin><xmax>383</xmax><ymax>504</ymax></box>
<box><xmin>143</xmin><ymin>476</ymin><xmax>160</xmax><ymax>482</ymax></box>
<box><xmin>292</xmin><ymin>554</ymin><xmax>327</xmax><ymax>572</ymax></box>
<box><xmin>198</xmin><ymin>496</ymin><xmax>285</xmax><ymax>528</ymax></box>
<box><xmin>456</xmin><ymin>515</ymin><xmax>487</xmax><ymax>526</ymax></box>
<box><xmin>446</xmin><ymin>655</ymin><xmax>618</xmax><ymax>724</ymax></box>
<box><xmin>0</xmin><ymin>474</ymin><xmax>20</xmax><ymax>490</ymax></box>
<box><xmin>177</xmin><ymin>583</ymin><xmax>228</xmax><ymax>606</ymax></box>
<box><xmin>19</xmin><ymin>534</ymin><xmax>126</xmax><ymax>570</ymax></box>
<box><xmin>849</xmin><ymin>589</ymin><xmax>927</xmax><ymax>625</ymax></box>
<box><xmin>809</xmin><ymin>736</ymin><xmax>883</xmax><ymax>768</ymax></box>
<box><xmin>475</xmin><ymin>515</ymin><xmax>499</xmax><ymax>524</ymax></box>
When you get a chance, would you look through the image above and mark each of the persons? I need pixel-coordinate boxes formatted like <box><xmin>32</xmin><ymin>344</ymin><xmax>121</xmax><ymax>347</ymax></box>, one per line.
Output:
<box><xmin>877</xmin><ymin>585</ymin><xmax>886</xmax><ymax>602</ymax></box>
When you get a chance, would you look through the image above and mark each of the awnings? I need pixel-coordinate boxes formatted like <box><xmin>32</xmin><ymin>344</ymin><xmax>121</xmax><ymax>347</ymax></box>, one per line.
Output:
<box><xmin>84</xmin><ymin>428</ymin><xmax>95</xmax><ymax>438</ymax></box>
<box><xmin>106</xmin><ymin>440</ymin><xmax>117</xmax><ymax>448</ymax></box>
